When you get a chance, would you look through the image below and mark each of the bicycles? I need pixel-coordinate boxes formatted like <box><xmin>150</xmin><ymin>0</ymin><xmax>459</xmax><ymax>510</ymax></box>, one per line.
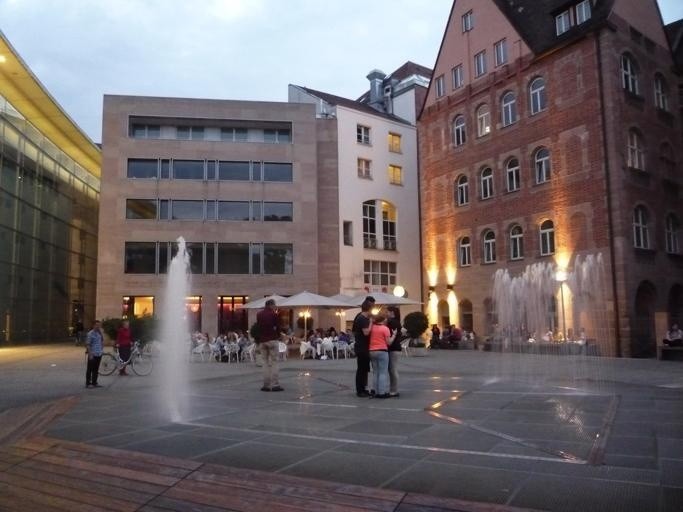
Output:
<box><xmin>96</xmin><ymin>339</ymin><xmax>154</xmax><ymax>376</ymax></box>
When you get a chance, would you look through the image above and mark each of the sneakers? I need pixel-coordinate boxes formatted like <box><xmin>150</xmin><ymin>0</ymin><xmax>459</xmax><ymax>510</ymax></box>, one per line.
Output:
<box><xmin>85</xmin><ymin>371</ymin><xmax>130</xmax><ymax>388</ymax></box>
<box><xmin>356</xmin><ymin>390</ymin><xmax>400</xmax><ymax>400</ymax></box>
<box><xmin>260</xmin><ymin>386</ymin><xmax>285</xmax><ymax>392</ymax></box>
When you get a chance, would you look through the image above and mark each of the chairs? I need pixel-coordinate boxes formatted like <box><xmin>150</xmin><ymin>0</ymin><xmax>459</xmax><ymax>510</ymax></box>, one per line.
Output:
<box><xmin>190</xmin><ymin>333</ymin><xmax>353</xmax><ymax>364</ymax></box>
<box><xmin>401</xmin><ymin>337</ymin><xmax>412</xmax><ymax>359</ymax></box>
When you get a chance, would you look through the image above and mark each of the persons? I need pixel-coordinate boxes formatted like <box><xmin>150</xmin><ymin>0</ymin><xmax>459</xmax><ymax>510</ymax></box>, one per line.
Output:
<box><xmin>116</xmin><ymin>318</ymin><xmax>135</xmax><ymax>378</ymax></box>
<box><xmin>186</xmin><ymin>323</ymin><xmax>466</xmax><ymax>355</ymax></box>
<box><xmin>660</xmin><ymin>322</ymin><xmax>683</xmax><ymax>347</ymax></box>
<box><xmin>253</xmin><ymin>298</ymin><xmax>284</xmax><ymax>392</ymax></box>
<box><xmin>489</xmin><ymin>319</ymin><xmax>587</xmax><ymax>347</ymax></box>
<box><xmin>353</xmin><ymin>294</ymin><xmax>401</xmax><ymax>398</ymax></box>
<box><xmin>83</xmin><ymin>318</ymin><xmax>108</xmax><ymax>389</ymax></box>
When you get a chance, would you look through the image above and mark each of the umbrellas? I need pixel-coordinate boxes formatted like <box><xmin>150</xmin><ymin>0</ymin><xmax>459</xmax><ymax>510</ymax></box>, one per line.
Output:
<box><xmin>241</xmin><ymin>291</ymin><xmax>287</xmax><ymax>310</ymax></box>
<box><xmin>324</xmin><ymin>294</ymin><xmax>354</xmax><ymax>306</ymax></box>
<box><xmin>352</xmin><ymin>291</ymin><xmax>424</xmax><ymax>304</ymax></box>
<box><xmin>276</xmin><ymin>291</ymin><xmax>353</xmax><ymax>341</ymax></box>
